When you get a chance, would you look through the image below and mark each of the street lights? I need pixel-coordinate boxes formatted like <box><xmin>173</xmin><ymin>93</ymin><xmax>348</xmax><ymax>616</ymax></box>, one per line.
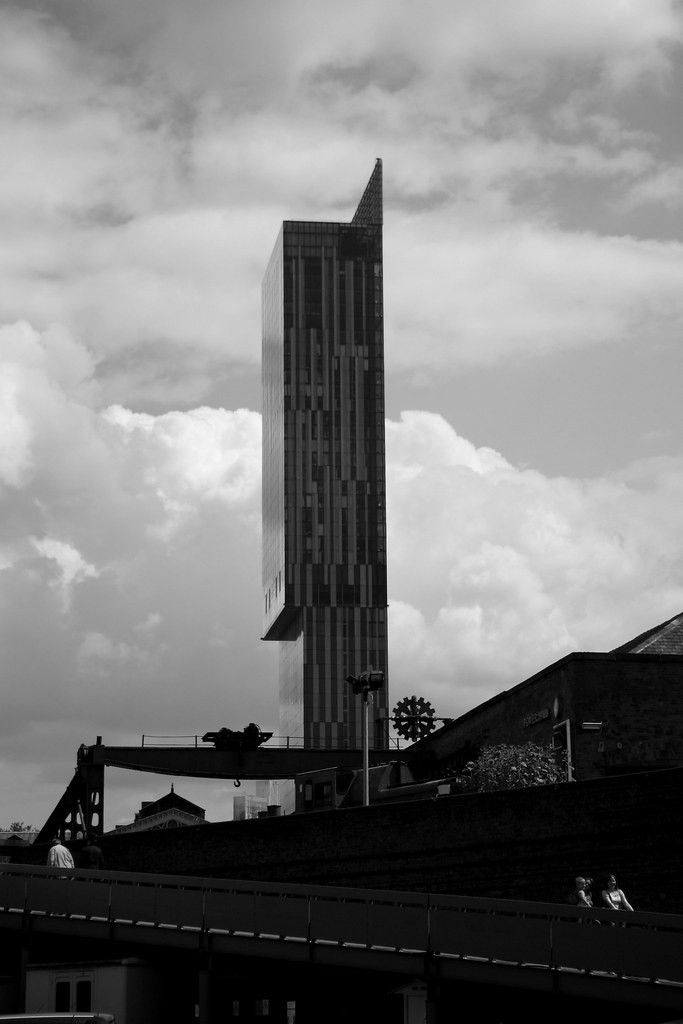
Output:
<box><xmin>345</xmin><ymin>671</ymin><xmax>386</xmax><ymax>806</ymax></box>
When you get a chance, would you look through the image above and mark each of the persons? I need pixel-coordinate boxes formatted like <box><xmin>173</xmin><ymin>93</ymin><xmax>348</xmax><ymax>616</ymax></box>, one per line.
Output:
<box><xmin>78</xmin><ymin>836</ymin><xmax>104</xmax><ymax>870</ymax></box>
<box><xmin>46</xmin><ymin>839</ymin><xmax>75</xmax><ymax>881</ymax></box>
<box><xmin>573</xmin><ymin>875</ymin><xmax>633</xmax><ymax>928</ymax></box>
